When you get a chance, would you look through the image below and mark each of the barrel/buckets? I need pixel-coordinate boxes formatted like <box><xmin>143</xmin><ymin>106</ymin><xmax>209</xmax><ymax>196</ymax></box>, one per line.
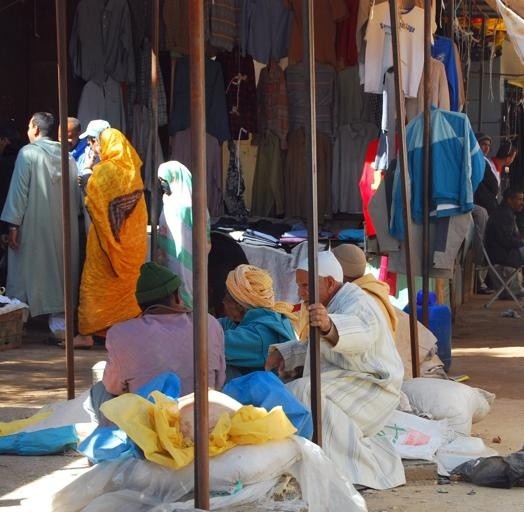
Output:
<box><xmin>402</xmin><ymin>304</ymin><xmax>452</xmax><ymax>373</ymax></box>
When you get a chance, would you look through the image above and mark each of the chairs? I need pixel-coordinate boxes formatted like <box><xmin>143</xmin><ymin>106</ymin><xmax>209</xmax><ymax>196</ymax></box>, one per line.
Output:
<box><xmin>476</xmin><ymin>224</ymin><xmax>524</xmax><ymax>311</ymax></box>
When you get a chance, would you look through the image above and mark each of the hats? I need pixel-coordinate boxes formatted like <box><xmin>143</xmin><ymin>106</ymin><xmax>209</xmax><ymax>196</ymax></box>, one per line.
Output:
<box><xmin>79</xmin><ymin>119</ymin><xmax>110</xmax><ymax>139</ymax></box>
<box><xmin>136</xmin><ymin>262</ymin><xmax>180</xmax><ymax>303</ymax></box>
<box><xmin>297</xmin><ymin>244</ymin><xmax>365</xmax><ymax>282</ymax></box>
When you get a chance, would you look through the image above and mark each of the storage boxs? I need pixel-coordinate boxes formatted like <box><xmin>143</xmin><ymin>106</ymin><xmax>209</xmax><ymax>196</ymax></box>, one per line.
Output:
<box><xmin>0</xmin><ymin>309</ymin><xmax>25</xmax><ymax>350</ymax></box>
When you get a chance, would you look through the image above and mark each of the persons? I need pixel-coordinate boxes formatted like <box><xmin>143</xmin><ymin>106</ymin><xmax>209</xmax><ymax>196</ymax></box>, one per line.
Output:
<box><xmin>154</xmin><ymin>160</ymin><xmax>212</xmax><ymax>309</ymax></box>
<box><xmin>90</xmin><ymin>263</ymin><xmax>226</xmax><ymax>424</ymax></box>
<box><xmin>55</xmin><ymin>127</ymin><xmax>148</xmax><ymax>347</ymax></box>
<box><xmin>1</xmin><ymin>111</ymin><xmax>86</xmax><ymax>340</ymax></box>
<box><xmin>78</xmin><ymin>119</ymin><xmax>111</xmax><ymax>189</ymax></box>
<box><xmin>265</xmin><ymin>250</ymin><xmax>407</xmax><ymax>492</ymax></box>
<box><xmin>218</xmin><ymin>264</ymin><xmax>296</xmax><ymax>380</ymax></box>
<box><xmin>473</xmin><ymin>131</ymin><xmax>524</xmax><ymax>295</ymax></box>
<box><xmin>297</xmin><ymin>244</ymin><xmax>397</xmax><ymax>341</ymax></box>
